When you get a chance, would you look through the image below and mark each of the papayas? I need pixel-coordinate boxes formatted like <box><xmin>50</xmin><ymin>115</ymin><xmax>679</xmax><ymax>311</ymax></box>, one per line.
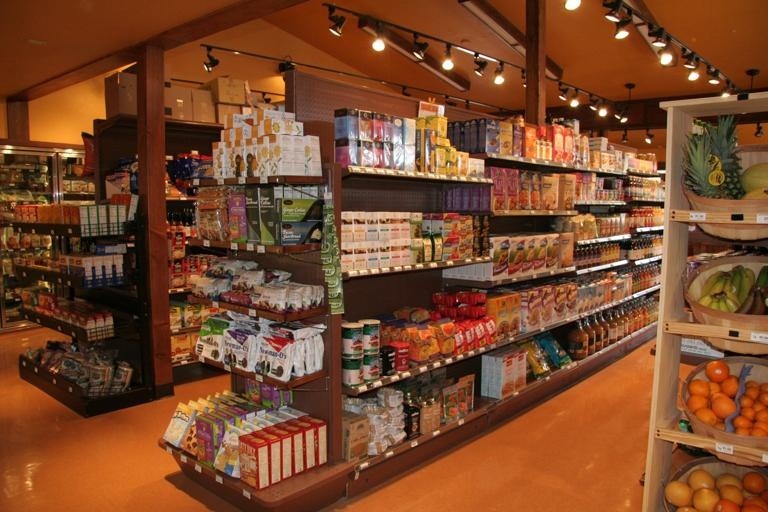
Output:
<box><xmin>738</xmin><ymin>162</ymin><xmax>768</xmax><ymax>201</ymax></box>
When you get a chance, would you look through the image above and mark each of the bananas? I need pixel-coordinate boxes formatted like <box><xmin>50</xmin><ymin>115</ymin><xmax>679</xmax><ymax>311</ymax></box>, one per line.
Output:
<box><xmin>694</xmin><ymin>263</ymin><xmax>768</xmax><ymax>315</ymax></box>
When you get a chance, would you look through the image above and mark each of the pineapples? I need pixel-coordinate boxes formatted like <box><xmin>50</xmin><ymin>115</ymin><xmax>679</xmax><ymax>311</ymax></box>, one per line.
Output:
<box><xmin>681</xmin><ymin>112</ymin><xmax>746</xmax><ymax>200</ymax></box>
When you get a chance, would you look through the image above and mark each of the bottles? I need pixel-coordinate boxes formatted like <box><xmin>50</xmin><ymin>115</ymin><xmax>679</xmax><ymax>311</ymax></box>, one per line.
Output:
<box><xmin>636</xmin><ymin>152</ymin><xmax>657</xmax><ymax>173</ymax></box>
<box><xmin>569</xmin><ymin>299</ymin><xmax>658</xmax><ymax>362</ymax></box>
<box><xmin>572</xmin><ymin>242</ymin><xmax>620</xmax><ymax>271</ymax></box>
<box><xmin>626</xmin><ymin>204</ymin><xmax>663</xmax><ymax>228</ymax></box>
<box><xmin>595</xmin><ymin>212</ymin><xmax>628</xmax><ymax>237</ymax></box>
<box><xmin>622</xmin><ymin>174</ymin><xmax>658</xmax><ymax>199</ymax></box>
<box><xmin>165</xmin><ymin>206</ymin><xmax>196</xmax><ymax>239</ymax></box>
<box><xmin>621</xmin><ymin>235</ymin><xmax>663</xmax><ymax>262</ymax></box>
<box><xmin>622</xmin><ymin>264</ymin><xmax>660</xmax><ymax>294</ymax></box>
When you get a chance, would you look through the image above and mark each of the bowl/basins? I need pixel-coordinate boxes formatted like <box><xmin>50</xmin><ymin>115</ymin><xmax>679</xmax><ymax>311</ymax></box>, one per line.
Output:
<box><xmin>680</xmin><ymin>141</ymin><xmax>768</xmax><ymax>240</ymax></box>
<box><xmin>681</xmin><ymin>355</ymin><xmax>768</xmax><ymax>469</ymax></box>
<box><xmin>662</xmin><ymin>457</ymin><xmax>768</xmax><ymax>511</ymax></box>
<box><xmin>681</xmin><ymin>255</ymin><xmax>767</xmax><ymax>356</ymax></box>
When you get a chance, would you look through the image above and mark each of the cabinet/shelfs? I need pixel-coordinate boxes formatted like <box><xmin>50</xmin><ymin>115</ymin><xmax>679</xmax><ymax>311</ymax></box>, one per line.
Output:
<box><xmin>0</xmin><ymin>139</ymin><xmax>95</xmax><ymax>333</ymax></box>
<box><xmin>13</xmin><ymin>112</ymin><xmax>228</xmax><ymax>418</ymax></box>
<box><xmin>158</xmin><ymin>120</ymin><xmax>665</xmax><ymax>512</ymax></box>
<box><xmin>641</xmin><ymin>92</ymin><xmax>768</xmax><ymax>512</ymax></box>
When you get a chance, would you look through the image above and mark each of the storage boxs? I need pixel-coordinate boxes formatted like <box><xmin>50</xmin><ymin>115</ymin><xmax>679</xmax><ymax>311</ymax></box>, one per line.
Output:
<box><xmin>104</xmin><ymin>72</ymin><xmax>254</xmax><ymax>123</ymax></box>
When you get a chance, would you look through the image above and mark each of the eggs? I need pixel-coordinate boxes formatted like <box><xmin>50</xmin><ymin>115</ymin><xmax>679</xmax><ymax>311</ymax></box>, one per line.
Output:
<box><xmin>686</xmin><ymin>359</ymin><xmax>768</xmax><ymax>438</ymax></box>
<box><xmin>660</xmin><ymin>470</ymin><xmax>768</xmax><ymax>512</ymax></box>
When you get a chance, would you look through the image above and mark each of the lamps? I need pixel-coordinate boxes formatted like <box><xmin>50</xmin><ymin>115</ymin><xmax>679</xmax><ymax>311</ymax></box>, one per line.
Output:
<box><xmin>277</xmin><ymin>61</ymin><xmax>297</xmax><ymax>73</ymax></box>
<box><xmin>564</xmin><ymin>0</ymin><xmax>734</xmax><ymax>96</ymax></box>
<box><xmin>202</xmin><ymin>60</ymin><xmax>217</xmax><ymax>70</ymax></box>
<box><xmin>328</xmin><ymin>17</ymin><xmax>653</xmax><ymax>145</ymax></box>
